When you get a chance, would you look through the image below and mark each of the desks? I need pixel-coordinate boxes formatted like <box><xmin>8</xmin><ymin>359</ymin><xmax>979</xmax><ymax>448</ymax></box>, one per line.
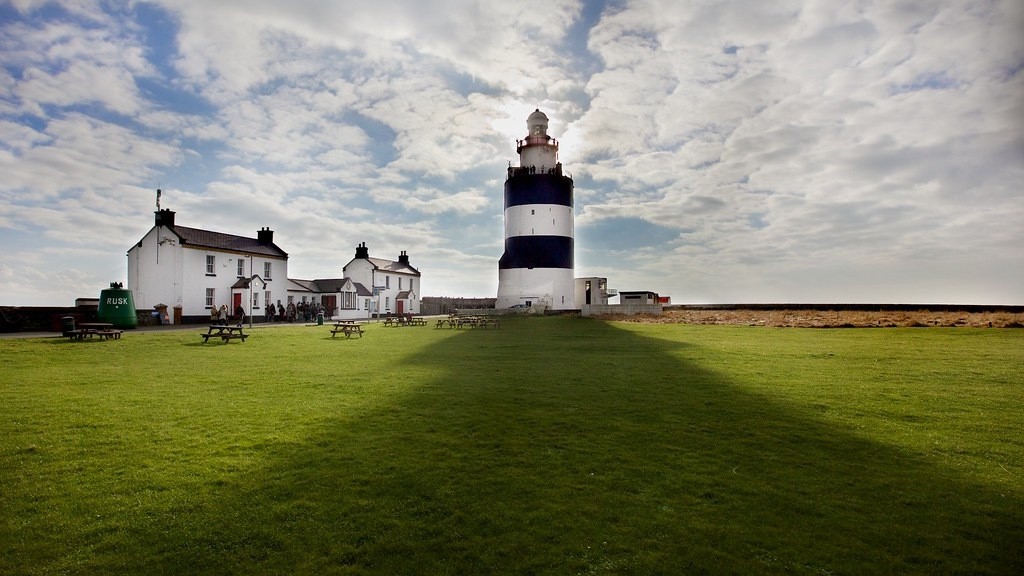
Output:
<box><xmin>460</xmin><ymin>315</ymin><xmax>472</xmax><ymax>318</ymax></box>
<box><xmin>482</xmin><ymin>319</ymin><xmax>499</xmax><ymax>328</ymax></box>
<box><xmin>473</xmin><ymin>314</ymin><xmax>489</xmax><ymax>319</ymax></box>
<box><xmin>438</xmin><ymin>319</ymin><xmax>455</xmax><ymax>328</ymax></box>
<box><xmin>412</xmin><ymin>317</ymin><xmax>423</xmax><ymax>325</ymax></box>
<box><xmin>338</xmin><ymin>319</ymin><xmax>354</xmax><ymax>324</ymax></box>
<box><xmin>79</xmin><ymin>322</ymin><xmax>113</xmax><ymax>340</ymax></box>
<box><xmin>332</xmin><ymin>324</ymin><xmax>362</xmax><ymax>339</ymax></box>
<box><xmin>204</xmin><ymin>325</ymin><xmax>245</xmax><ymax>343</ymax></box>
<box><xmin>387</xmin><ymin>317</ymin><xmax>399</xmax><ymax>326</ymax></box>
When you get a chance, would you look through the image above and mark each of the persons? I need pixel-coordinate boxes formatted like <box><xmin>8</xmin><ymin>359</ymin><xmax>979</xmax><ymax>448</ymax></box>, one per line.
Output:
<box><xmin>265</xmin><ymin>301</ymin><xmax>338</xmax><ymax>322</ymax></box>
<box><xmin>210</xmin><ymin>304</ymin><xmax>228</xmax><ymax>326</ymax></box>
<box><xmin>236</xmin><ymin>303</ymin><xmax>246</xmax><ymax>327</ymax></box>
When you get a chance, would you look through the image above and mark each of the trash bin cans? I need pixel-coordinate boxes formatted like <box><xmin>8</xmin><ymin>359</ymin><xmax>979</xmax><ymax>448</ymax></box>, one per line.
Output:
<box><xmin>61</xmin><ymin>316</ymin><xmax>75</xmax><ymax>337</ymax></box>
<box><xmin>317</xmin><ymin>313</ymin><xmax>323</xmax><ymax>325</ymax></box>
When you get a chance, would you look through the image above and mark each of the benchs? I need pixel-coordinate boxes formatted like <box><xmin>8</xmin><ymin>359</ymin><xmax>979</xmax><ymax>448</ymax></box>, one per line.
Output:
<box><xmin>345</xmin><ymin>329</ymin><xmax>364</xmax><ymax>333</ymax></box>
<box><xmin>330</xmin><ymin>330</ymin><xmax>353</xmax><ymax>334</ymax></box>
<box><xmin>101</xmin><ymin>329</ymin><xmax>124</xmax><ymax>339</ymax></box>
<box><xmin>97</xmin><ymin>331</ymin><xmax>119</xmax><ymax>340</ymax></box>
<box><xmin>225</xmin><ymin>333</ymin><xmax>249</xmax><ymax>337</ymax></box>
<box><xmin>67</xmin><ymin>329</ymin><xmax>95</xmax><ymax>339</ymax></box>
<box><xmin>384</xmin><ymin>320</ymin><xmax>500</xmax><ymax>329</ymax></box>
<box><xmin>199</xmin><ymin>333</ymin><xmax>231</xmax><ymax>338</ymax></box>
